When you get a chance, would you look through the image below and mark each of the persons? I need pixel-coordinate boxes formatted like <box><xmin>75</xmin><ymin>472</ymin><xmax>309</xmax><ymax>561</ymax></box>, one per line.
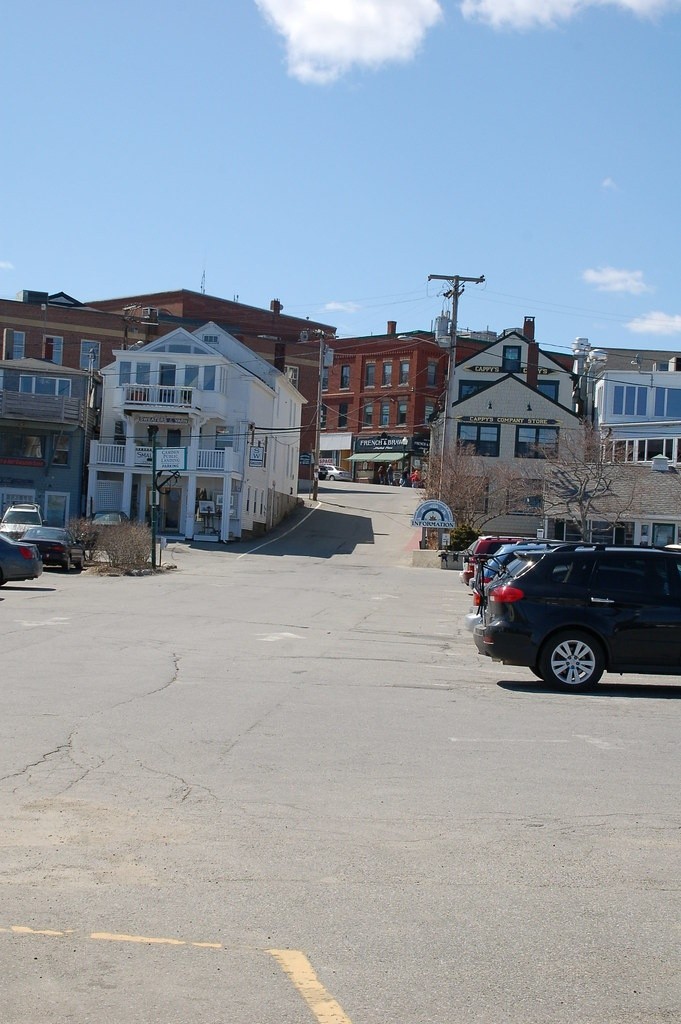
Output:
<box><xmin>377</xmin><ymin>463</ymin><xmax>422</xmax><ymax>487</ymax></box>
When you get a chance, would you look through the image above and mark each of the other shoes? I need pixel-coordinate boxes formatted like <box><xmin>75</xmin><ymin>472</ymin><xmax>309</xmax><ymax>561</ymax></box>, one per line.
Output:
<box><xmin>399</xmin><ymin>485</ymin><xmax>402</xmax><ymax>487</ymax></box>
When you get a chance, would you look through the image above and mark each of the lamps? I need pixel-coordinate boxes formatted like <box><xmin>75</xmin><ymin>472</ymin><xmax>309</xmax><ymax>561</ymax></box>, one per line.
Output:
<box><xmin>527</xmin><ymin>401</ymin><xmax>532</xmax><ymax>411</ymax></box>
<box><xmin>488</xmin><ymin>401</ymin><xmax>492</xmax><ymax>409</ymax></box>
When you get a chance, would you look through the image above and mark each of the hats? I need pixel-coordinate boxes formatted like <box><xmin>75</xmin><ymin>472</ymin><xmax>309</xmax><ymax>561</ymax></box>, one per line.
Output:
<box><xmin>388</xmin><ymin>464</ymin><xmax>392</xmax><ymax>467</ymax></box>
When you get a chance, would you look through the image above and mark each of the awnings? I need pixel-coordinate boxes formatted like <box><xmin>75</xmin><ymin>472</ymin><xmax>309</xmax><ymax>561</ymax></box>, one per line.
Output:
<box><xmin>346</xmin><ymin>452</ymin><xmax>409</xmax><ymax>463</ymax></box>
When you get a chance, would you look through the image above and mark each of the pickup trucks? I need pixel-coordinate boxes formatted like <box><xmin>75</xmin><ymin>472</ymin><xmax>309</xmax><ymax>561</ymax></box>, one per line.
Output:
<box><xmin>0</xmin><ymin>503</ymin><xmax>49</xmax><ymax>541</ymax></box>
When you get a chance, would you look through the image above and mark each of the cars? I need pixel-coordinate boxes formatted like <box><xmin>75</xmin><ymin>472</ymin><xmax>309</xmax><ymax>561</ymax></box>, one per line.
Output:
<box><xmin>0</xmin><ymin>531</ymin><xmax>43</xmax><ymax>586</ymax></box>
<box><xmin>319</xmin><ymin>465</ymin><xmax>352</xmax><ymax>481</ymax></box>
<box><xmin>17</xmin><ymin>527</ymin><xmax>84</xmax><ymax>572</ymax></box>
<box><xmin>91</xmin><ymin>510</ymin><xmax>131</xmax><ymax>527</ymax></box>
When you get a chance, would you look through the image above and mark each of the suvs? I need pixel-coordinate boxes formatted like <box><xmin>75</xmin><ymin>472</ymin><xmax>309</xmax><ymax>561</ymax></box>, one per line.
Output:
<box><xmin>458</xmin><ymin>533</ymin><xmax>681</xmax><ymax>692</ymax></box>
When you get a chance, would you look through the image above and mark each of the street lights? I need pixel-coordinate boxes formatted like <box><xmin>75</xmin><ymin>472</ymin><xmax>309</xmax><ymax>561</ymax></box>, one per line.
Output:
<box><xmin>401</xmin><ymin>437</ymin><xmax>409</xmax><ymax>470</ymax></box>
